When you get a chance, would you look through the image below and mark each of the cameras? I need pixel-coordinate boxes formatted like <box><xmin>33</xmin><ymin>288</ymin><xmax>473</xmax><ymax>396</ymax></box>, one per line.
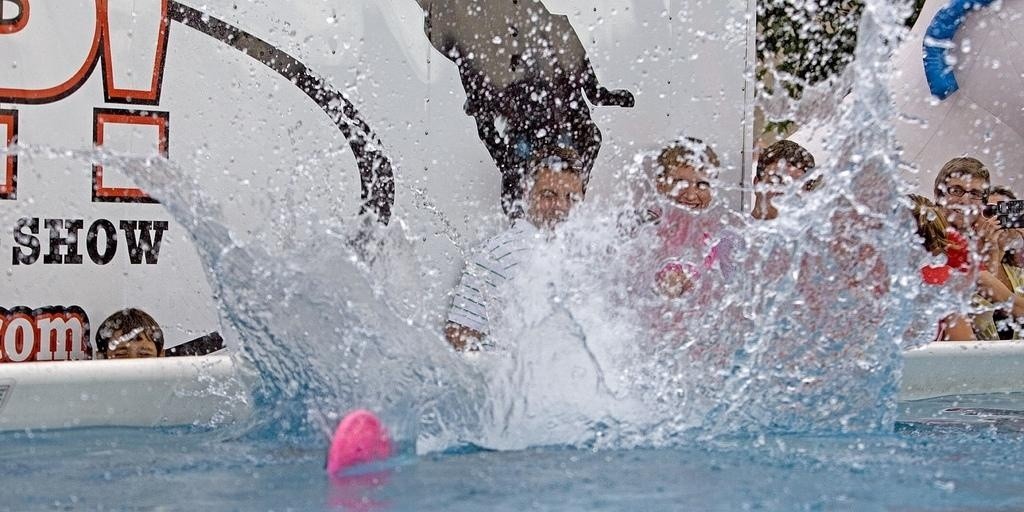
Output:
<box><xmin>983</xmin><ymin>199</ymin><xmax>1024</xmax><ymax>229</ymax></box>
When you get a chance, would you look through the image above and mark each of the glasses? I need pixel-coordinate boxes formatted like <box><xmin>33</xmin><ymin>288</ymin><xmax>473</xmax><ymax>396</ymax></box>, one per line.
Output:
<box><xmin>947</xmin><ymin>186</ymin><xmax>986</xmax><ymax>200</ymax></box>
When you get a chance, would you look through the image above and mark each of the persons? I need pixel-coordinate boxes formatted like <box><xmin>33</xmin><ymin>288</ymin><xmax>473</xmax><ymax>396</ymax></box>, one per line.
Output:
<box><xmin>443</xmin><ymin>136</ymin><xmax>1023</xmax><ymax>351</ymax></box>
<box><xmin>95</xmin><ymin>308</ymin><xmax>165</xmax><ymax>359</ymax></box>
